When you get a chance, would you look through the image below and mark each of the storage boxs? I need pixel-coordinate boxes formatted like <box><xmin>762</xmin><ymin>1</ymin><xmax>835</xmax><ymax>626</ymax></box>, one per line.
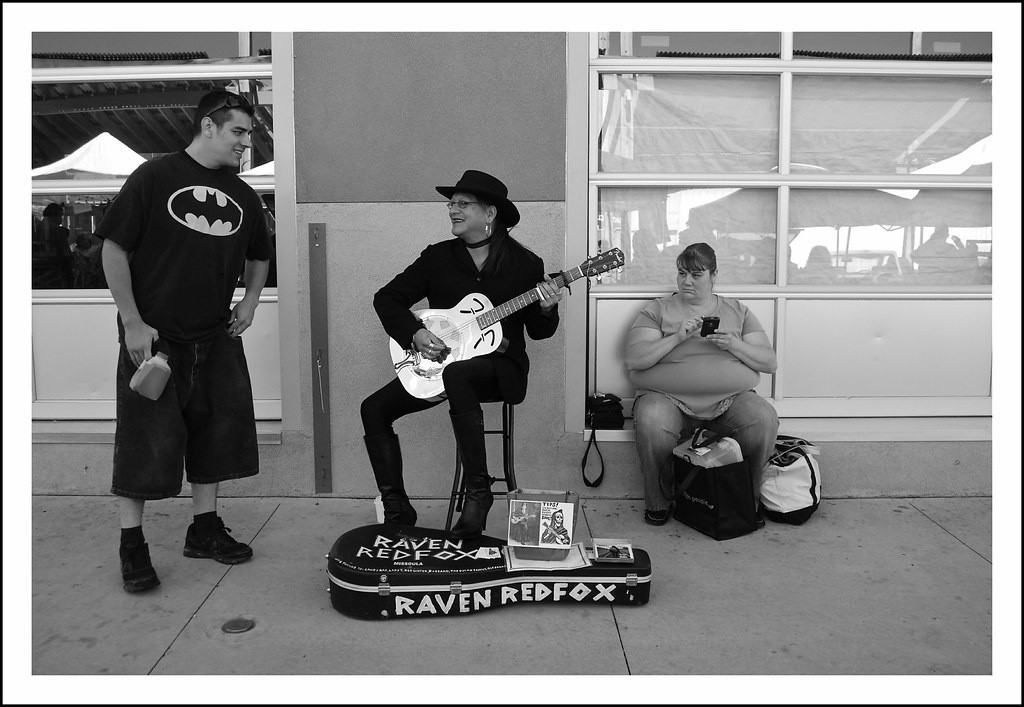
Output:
<box><xmin>671</xmin><ymin>428</ymin><xmax>744</xmax><ymax>470</ymax></box>
<box><xmin>325</xmin><ymin>521</ymin><xmax>653</xmax><ymax>621</ymax></box>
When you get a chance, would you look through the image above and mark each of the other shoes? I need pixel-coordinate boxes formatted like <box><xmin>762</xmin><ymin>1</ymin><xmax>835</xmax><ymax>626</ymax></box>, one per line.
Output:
<box><xmin>646</xmin><ymin>503</ymin><xmax>671</xmax><ymax>525</ymax></box>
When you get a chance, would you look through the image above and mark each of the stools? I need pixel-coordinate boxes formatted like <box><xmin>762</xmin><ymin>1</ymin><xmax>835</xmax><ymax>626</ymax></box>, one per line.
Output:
<box><xmin>444</xmin><ymin>391</ymin><xmax>518</xmax><ymax>533</ymax></box>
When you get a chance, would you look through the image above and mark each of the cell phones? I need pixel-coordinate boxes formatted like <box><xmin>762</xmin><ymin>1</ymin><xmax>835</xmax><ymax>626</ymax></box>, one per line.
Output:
<box><xmin>700</xmin><ymin>317</ymin><xmax>720</xmax><ymax>337</ymax></box>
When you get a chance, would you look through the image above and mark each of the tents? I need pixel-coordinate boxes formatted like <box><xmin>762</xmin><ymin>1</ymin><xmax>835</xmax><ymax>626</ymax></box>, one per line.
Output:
<box><xmin>32</xmin><ymin>131</ymin><xmax>275</xmax><ymax>234</ymax></box>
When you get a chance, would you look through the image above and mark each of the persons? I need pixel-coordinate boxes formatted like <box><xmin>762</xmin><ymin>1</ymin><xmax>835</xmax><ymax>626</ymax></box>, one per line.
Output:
<box><xmin>361</xmin><ymin>170</ymin><xmax>563</xmax><ymax>543</ymax></box>
<box><xmin>103</xmin><ymin>95</ymin><xmax>273</xmax><ymax>595</ymax></box>
<box><xmin>623</xmin><ymin>243</ymin><xmax>779</xmax><ymax>525</ymax></box>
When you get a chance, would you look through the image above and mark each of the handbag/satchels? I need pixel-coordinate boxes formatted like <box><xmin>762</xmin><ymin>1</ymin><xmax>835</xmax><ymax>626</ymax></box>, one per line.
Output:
<box><xmin>581</xmin><ymin>393</ymin><xmax>624</xmax><ymax>488</ymax></box>
<box><xmin>658</xmin><ymin>415</ymin><xmax>756</xmax><ymax>541</ymax></box>
<box><xmin>761</xmin><ymin>436</ymin><xmax>821</xmax><ymax>526</ymax></box>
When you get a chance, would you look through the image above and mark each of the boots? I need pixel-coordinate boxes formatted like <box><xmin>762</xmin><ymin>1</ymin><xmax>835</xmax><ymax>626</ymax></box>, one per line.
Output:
<box><xmin>362</xmin><ymin>432</ymin><xmax>416</xmax><ymax>528</ymax></box>
<box><xmin>449</xmin><ymin>406</ymin><xmax>493</xmax><ymax>540</ymax></box>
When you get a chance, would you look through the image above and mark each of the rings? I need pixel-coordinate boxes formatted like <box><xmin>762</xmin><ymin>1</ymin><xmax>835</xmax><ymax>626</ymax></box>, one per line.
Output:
<box><xmin>423</xmin><ymin>343</ymin><xmax>434</xmax><ymax>358</ymax></box>
<box><xmin>541</xmin><ymin>291</ymin><xmax>561</xmax><ymax>301</ymax></box>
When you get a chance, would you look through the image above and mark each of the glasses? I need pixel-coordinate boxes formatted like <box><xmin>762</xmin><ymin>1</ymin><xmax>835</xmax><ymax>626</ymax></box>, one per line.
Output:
<box><xmin>204</xmin><ymin>94</ymin><xmax>252</xmax><ymax>116</ymax></box>
<box><xmin>447</xmin><ymin>197</ymin><xmax>491</xmax><ymax>210</ymax></box>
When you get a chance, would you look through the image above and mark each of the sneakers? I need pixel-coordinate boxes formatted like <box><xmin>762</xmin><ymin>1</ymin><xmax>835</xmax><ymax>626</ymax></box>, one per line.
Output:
<box><xmin>119</xmin><ymin>536</ymin><xmax>159</xmax><ymax>592</ymax></box>
<box><xmin>182</xmin><ymin>515</ymin><xmax>254</xmax><ymax>565</ymax></box>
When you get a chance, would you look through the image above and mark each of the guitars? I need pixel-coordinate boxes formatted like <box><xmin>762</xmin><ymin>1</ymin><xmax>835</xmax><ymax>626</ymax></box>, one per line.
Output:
<box><xmin>388</xmin><ymin>245</ymin><xmax>628</xmax><ymax>405</ymax></box>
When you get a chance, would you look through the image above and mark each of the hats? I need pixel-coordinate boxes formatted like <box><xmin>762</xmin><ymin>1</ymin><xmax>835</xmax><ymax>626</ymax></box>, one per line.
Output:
<box><xmin>436</xmin><ymin>169</ymin><xmax>520</xmax><ymax>227</ymax></box>
